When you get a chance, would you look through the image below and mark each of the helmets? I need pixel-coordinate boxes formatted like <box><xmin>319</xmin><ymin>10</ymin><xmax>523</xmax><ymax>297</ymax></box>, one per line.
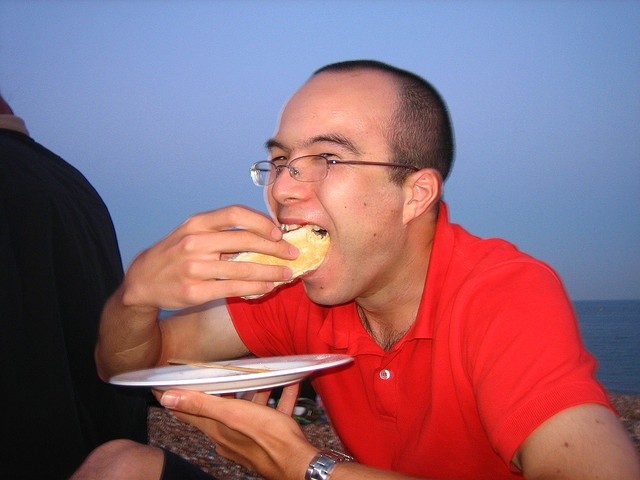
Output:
<box><xmin>106</xmin><ymin>354</ymin><xmax>354</xmax><ymax>403</ymax></box>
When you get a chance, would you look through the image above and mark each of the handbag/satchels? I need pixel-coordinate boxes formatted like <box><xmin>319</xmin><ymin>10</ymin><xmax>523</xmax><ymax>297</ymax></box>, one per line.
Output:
<box><xmin>304</xmin><ymin>449</ymin><xmax>354</xmax><ymax>479</ymax></box>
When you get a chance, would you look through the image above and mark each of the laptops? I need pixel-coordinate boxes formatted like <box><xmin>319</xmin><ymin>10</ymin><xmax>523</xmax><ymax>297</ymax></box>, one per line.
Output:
<box><xmin>227</xmin><ymin>226</ymin><xmax>332</xmax><ymax>301</ymax></box>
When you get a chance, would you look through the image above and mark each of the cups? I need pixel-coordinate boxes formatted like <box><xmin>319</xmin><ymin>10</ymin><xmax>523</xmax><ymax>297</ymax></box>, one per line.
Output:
<box><xmin>250</xmin><ymin>155</ymin><xmax>419</xmax><ymax>187</ymax></box>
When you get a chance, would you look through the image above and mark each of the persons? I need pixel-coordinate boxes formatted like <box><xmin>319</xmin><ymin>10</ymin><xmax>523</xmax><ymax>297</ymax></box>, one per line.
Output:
<box><xmin>72</xmin><ymin>60</ymin><xmax>640</xmax><ymax>479</ymax></box>
<box><xmin>0</xmin><ymin>94</ymin><xmax>147</xmax><ymax>480</ymax></box>
